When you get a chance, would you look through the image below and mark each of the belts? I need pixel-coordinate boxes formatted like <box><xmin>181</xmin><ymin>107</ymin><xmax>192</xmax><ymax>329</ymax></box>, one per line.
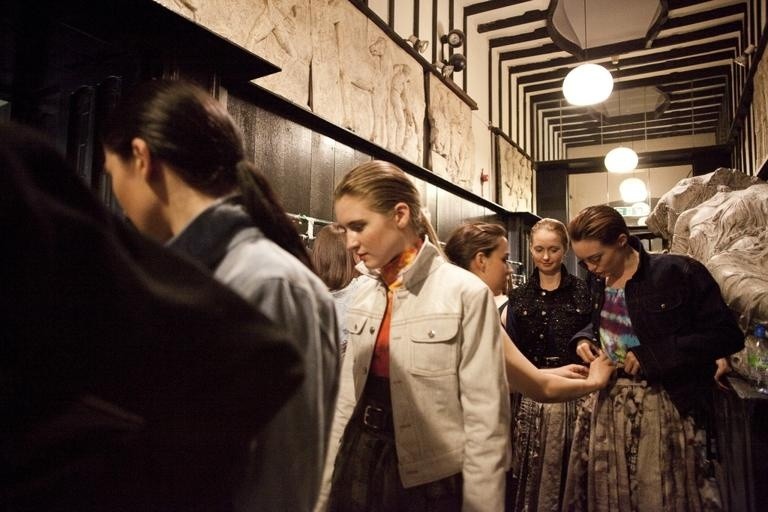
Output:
<box><xmin>352</xmin><ymin>403</ymin><xmax>394</xmax><ymax>433</ymax></box>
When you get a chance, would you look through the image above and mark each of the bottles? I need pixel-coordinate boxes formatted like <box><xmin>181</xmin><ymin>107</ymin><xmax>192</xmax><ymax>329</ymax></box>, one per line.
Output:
<box><xmin>748</xmin><ymin>327</ymin><xmax>768</xmax><ymax>397</ymax></box>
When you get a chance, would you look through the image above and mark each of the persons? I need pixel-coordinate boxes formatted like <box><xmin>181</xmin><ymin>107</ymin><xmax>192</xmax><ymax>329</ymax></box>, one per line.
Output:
<box><xmin>1</xmin><ymin>78</ymin><xmax>745</xmax><ymax>512</ymax></box>
